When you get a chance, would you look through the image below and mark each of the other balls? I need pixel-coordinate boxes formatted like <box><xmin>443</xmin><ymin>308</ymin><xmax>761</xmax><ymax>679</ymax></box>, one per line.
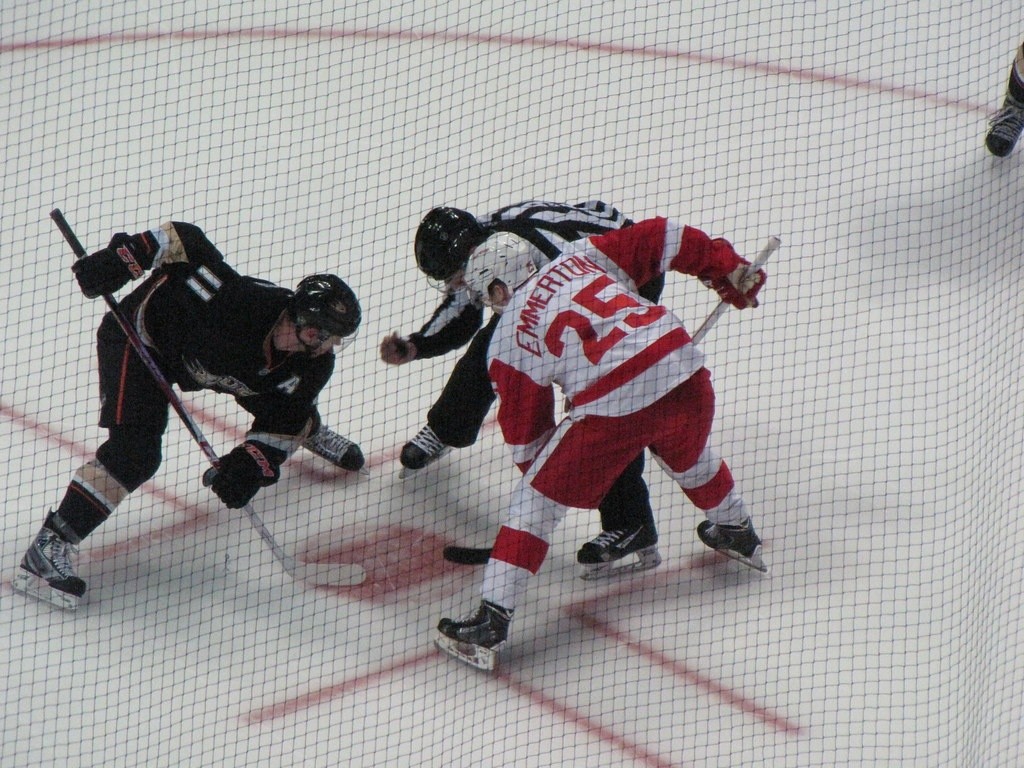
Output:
<box><xmin>388</xmin><ymin>338</ymin><xmax>407</xmax><ymax>358</ymax></box>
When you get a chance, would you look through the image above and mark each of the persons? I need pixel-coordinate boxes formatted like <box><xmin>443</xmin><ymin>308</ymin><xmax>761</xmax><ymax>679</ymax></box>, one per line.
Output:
<box><xmin>986</xmin><ymin>41</ymin><xmax>1024</xmax><ymax>158</ymax></box>
<box><xmin>10</xmin><ymin>221</ymin><xmax>370</xmax><ymax>609</ymax></box>
<box><xmin>381</xmin><ymin>201</ymin><xmax>766</xmax><ymax>672</ymax></box>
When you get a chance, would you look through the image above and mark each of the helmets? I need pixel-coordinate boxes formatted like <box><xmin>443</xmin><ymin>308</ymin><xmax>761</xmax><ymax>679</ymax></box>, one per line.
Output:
<box><xmin>289</xmin><ymin>274</ymin><xmax>362</xmax><ymax>338</ymax></box>
<box><xmin>414</xmin><ymin>206</ymin><xmax>480</xmax><ymax>282</ymax></box>
<box><xmin>464</xmin><ymin>230</ymin><xmax>538</xmax><ymax>309</ymax></box>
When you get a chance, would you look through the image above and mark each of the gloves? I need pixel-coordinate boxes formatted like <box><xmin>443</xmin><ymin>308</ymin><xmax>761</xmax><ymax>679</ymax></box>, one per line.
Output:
<box><xmin>71</xmin><ymin>233</ymin><xmax>151</xmax><ymax>300</ymax></box>
<box><xmin>202</xmin><ymin>439</ymin><xmax>288</xmax><ymax>510</ymax></box>
<box><xmin>380</xmin><ymin>332</ymin><xmax>417</xmax><ymax>367</ymax></box>
<box><xmin>698</xmin><ymin>239</ymin><xmax>767</xmax><ymax>310</ymax></box>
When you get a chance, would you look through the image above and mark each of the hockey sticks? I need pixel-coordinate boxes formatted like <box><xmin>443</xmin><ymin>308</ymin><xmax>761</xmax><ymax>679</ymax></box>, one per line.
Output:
<box><xmin>440</xmin><ymin>237</ymin><xmax>782</xmax><ymax>568</ymax></box>
<box><xmin>49</xmin><ymin>207</ymin><xmax>366</xmax><ymax>585</ymax></box>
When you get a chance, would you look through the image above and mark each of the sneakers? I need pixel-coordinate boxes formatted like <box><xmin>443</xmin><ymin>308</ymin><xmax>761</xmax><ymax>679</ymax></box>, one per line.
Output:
<box><xmin>11</xmin><ymin>506</ymin><xmax>87</xmax><ymax>612</ymax></box>
<box><xmin>983</xmin><ymin>60</ymin><xmax>1024</xmax><ymax>157</ymax></box>
<box><xmin>398</xmin><ymin>423</ymin><xmax>456</xmax><ymax>479</ymax></box>
<box><xmin>697</xmin><ymin>516</ymin><xmax>769</xmax><ymax>573</ymax></box>
<box><xmin>433</xmin><ymin>599</ymin><xmax>514</xmax><ymax>671</ymax></box>
<box><xmin>300</xmin><ymin>411</ymin><xmax>370</xmax><ymax>477</ymax></box>
<box><xmin>577</xmin><ymin>519</ymin><xmax>663</xmax><ymax>582</ymax></box>
